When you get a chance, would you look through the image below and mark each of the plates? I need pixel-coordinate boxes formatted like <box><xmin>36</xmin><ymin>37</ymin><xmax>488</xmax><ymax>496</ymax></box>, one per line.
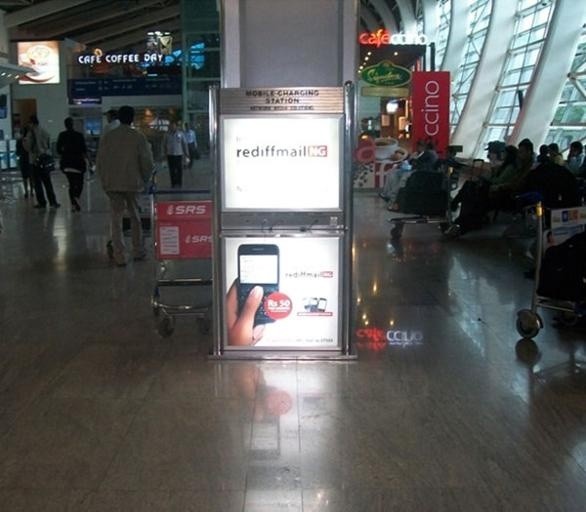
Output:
<box><xmin>373</xmin><ymin>146</ymin><xmax>408</xmax><ymax>164</ymax></box>
<box><xmin>18</xmin><ymin>56</ymin><xmax>58</xmax><ymax>81</ymax></box>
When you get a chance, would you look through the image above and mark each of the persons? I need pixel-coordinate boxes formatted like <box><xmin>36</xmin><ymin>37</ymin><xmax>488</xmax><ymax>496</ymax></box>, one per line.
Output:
<box><xmin>227</xmin><ymin>279</ymin><xmax>264</xmax><ymax>347</ymax></box>
<box><xmin>377</xmin><ymin>138</ymin><xmax>586</xmax><ymax>237</ymax></box>
<box><xmin>15</xmin><ymin>105</ymin><xmax>199</xmax><ymax>266</ymax></box>
<box><xmin>227</xmin><ymin>364</ymin><xmax>266</xmax><ymax>422</ymax></box>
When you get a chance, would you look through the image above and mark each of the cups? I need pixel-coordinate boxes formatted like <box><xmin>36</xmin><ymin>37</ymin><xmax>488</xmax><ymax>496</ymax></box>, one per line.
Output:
<box><xmin>372</xmin><ymin>137</ymin><xmax>398</xmax><ymax>159</ymax></box>
<box><xmin>21</xmin><ymin>46</ymin><xmax>54</xmax><ymax>72</ymax></box>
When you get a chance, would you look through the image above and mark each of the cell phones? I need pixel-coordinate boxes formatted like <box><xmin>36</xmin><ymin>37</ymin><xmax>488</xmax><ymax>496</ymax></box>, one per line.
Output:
<box><xmin>237</xmin><ymin>244</ymin><xmax>280</xmax><ymax>324</ymax></box>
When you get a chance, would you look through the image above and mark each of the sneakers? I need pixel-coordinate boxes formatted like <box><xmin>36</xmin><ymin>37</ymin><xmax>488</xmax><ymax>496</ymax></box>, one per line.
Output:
<box><xmin>30</xmin><ymin>202</ymin><xmax>61</xmax><ymax>209</ymax></box>
<box><xmin>70</xmin><ymin>197</ymin><xmax>82</xmax><ymax>212</ymax></box>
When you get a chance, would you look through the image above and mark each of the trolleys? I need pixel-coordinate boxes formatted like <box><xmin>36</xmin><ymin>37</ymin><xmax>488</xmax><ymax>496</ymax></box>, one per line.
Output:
<box><xmin>148</xmin><ymin>170</ymin><xmax>212</xmax><ymax>337</ymax></box>
<box><xmin>379</xmin><ymin>158</ymin><xmax>465</xmax><ymax>241</ymax></box>
<box><xmin>104</xmin><ymin>170</ymin><xmax>160</xmax><ymax>256</ymax></box>
<box><xmin>511</xmin><ymin>191</ymin><xmax>586</xmax><ymax>338</ymax></box>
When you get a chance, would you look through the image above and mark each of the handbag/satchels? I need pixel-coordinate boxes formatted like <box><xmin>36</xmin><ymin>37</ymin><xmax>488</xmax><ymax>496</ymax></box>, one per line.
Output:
<box><xmin>35</xmin><ymin>153</ymin><xmax>56</xmax><ymax>173</ymax></box>
